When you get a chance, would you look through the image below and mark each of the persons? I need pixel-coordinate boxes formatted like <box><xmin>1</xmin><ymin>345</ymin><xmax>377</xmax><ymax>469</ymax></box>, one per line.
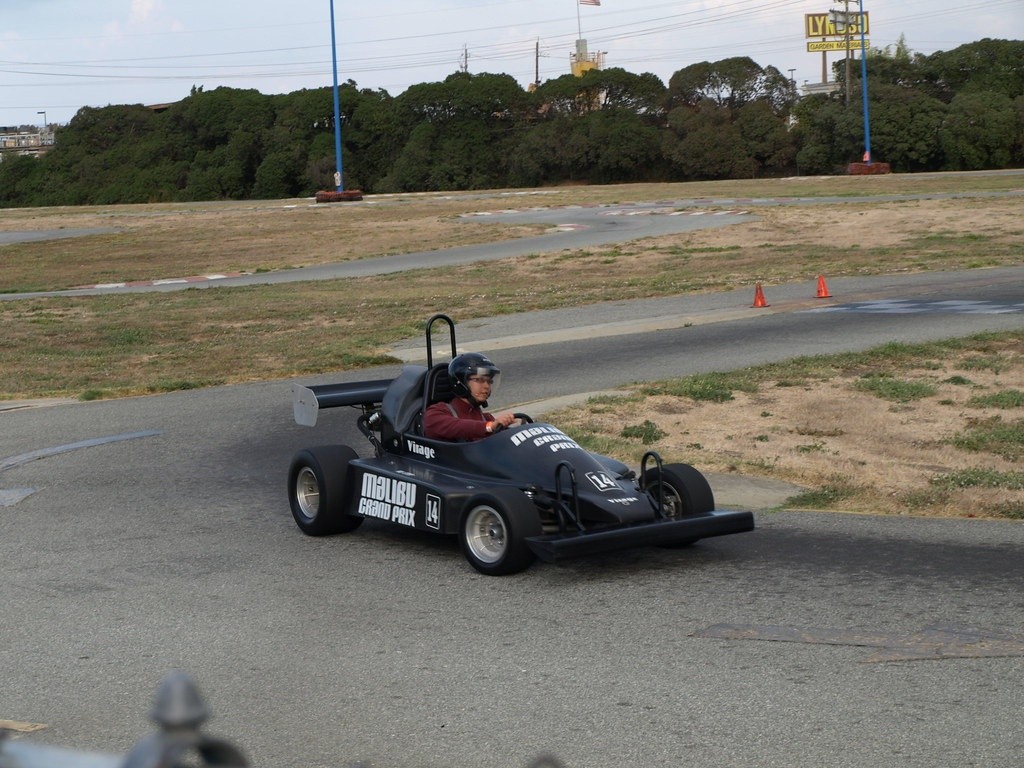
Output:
<box><xmin>422</xmin><ymin>352</ymin><xmax>517</xmax><ymax>442</ymax></box>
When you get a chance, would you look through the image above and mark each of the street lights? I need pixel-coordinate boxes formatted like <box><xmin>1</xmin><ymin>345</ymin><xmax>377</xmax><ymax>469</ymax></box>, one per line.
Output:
<box><xmin>788</xmin><ymin>69</ymin><xmax>797</xmax><ymax>109</ymax></box>
<box><xmin>38</xmin><ymin>111</ymin><xmax>48</xmax><ymax>130</ymax></box>
<box><xmin>601</xmin><ymin>52</ymin><xmax>607</xmax><ymax>70</ymax></box>
<box><xmin>805</xmin><ymin>79</ymin><xmax>808</xmax><ymax>91</ymax></box>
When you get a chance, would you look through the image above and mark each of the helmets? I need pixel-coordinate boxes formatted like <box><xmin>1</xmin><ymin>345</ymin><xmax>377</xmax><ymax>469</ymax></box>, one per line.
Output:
<box><xmin>448</xmin><ymin>352</ymin><xmax>501</xmax><ymax>399</ymax></box>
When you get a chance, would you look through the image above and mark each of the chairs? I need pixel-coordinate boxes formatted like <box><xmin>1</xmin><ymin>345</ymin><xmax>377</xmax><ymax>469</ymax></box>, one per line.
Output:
<box><xmin>414</xmin><ymin>361</ymin><xmax>463</xmax><ymax>440</ymax></box>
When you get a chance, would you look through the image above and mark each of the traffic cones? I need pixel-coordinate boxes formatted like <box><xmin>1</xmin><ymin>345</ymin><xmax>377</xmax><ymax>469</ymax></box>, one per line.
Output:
<box><xmin>816</xmin><ymin>275</ymin><xmax>833</xmax><ymax>297</ymax></box>
<box><xmin>752</xmin><ymin>282</ymin><xmax>770</xmax><ymax>308</ymax></box>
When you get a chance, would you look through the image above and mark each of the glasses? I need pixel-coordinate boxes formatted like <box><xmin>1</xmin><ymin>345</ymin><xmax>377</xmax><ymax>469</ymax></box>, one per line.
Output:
<box><xmin>469</xmin><ymin>378</ymin><xmax>493</xmax><ymax>385</ymax></box>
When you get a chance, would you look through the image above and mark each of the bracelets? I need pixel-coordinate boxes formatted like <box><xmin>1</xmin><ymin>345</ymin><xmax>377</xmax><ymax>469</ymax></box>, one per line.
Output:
<box><xmin>486</xmin><ymin>422</ymin><xmax>494</xmax><ymax>432</ymax></box>
